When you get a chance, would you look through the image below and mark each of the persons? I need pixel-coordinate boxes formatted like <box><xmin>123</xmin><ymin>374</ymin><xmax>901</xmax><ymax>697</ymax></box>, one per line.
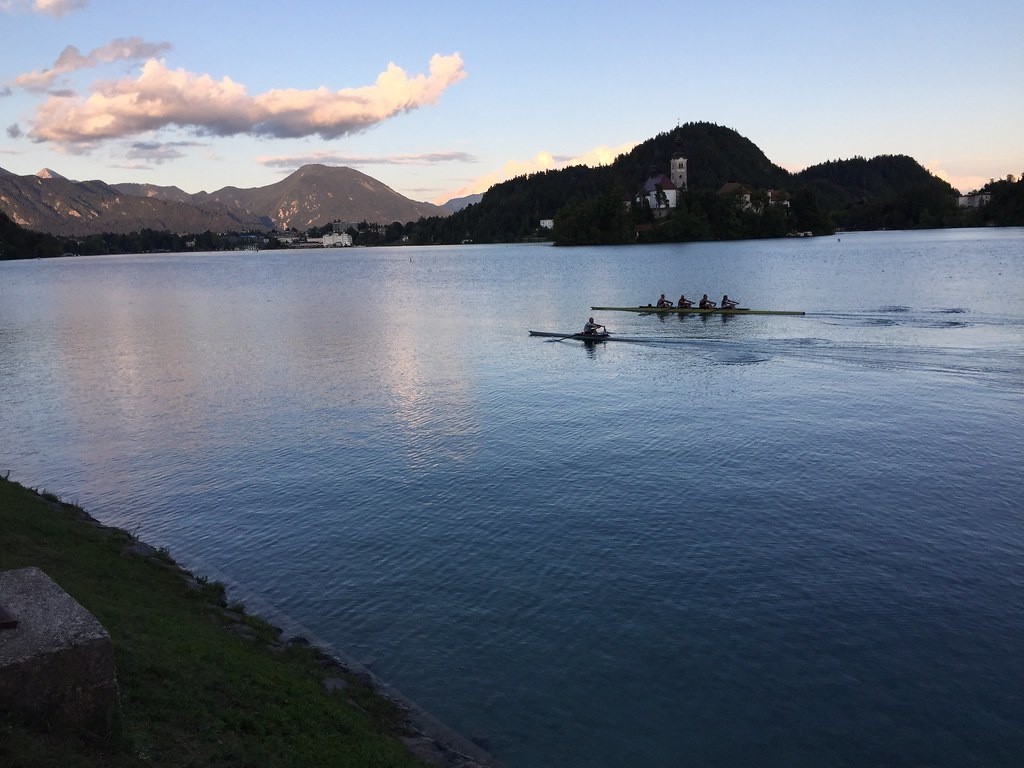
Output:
<box><xmin>699</xmin><ymin>294</ymin><xmax>716</xmax><ymax>308</ymax></box>
<box><xmin>657</xmin><ymin>294</ymin><xmax>673</xmax><ymax>308</ymax></box>
<box><xmin>722</xmin><ymin>295</ymin><xmax>738</xmax><ymax>309</ymax></box>
<box><xmin>584</xmin><ymin>318</ymin><xmax>600</xmax><ymax>334</ymax></box>
<box><xmin>677</xmin><ymin>295</ymin><xmax>695</xmax><ymax>307</ymax></box>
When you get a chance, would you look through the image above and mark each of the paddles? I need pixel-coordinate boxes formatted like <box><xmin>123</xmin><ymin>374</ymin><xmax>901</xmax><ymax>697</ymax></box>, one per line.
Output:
<box><xmin>554</xmin><ymin>332</ymin><xmax>585</xmax><ymax>342</ymax></box>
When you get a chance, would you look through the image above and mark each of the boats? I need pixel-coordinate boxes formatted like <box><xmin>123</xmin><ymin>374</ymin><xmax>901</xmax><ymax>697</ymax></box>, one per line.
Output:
<box><xmin>529</xmin><ymin>330</ymin><xmax>609</xmax><ymax>340</ymax></box>
<box><xmin>591</xmin><ymin>307</ymin><xmax>805</xmax><ymax>315</ymax></box>
